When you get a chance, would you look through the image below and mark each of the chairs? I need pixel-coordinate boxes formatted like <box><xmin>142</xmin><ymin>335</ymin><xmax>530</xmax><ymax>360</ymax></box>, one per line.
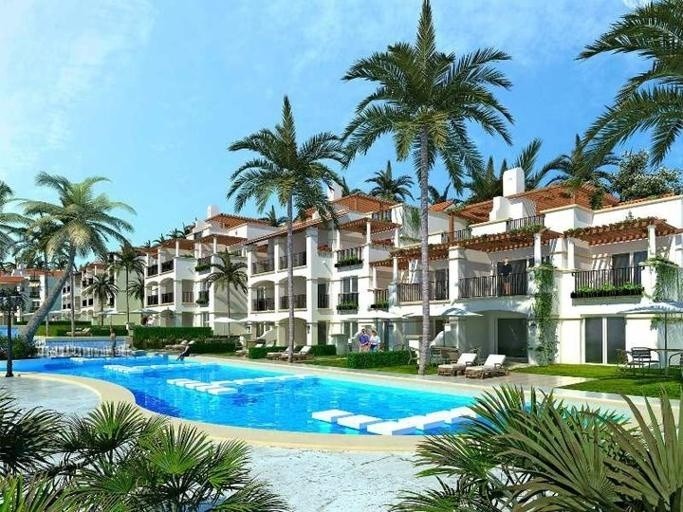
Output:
<box><xmin>66</xmin><ymin>328</ymin><xmax>90</xmax><ymax>335</ymax></box>
<box><xmin>616</xmin><ymin>346</ymin><xmax>683</xmax><ymax>377</ymax></box>
<box><xmin>266</xmin><ymin>346</ymin><xmax>311</xmax><ymax>362</ymax></box>
<box><xmin>404</xmin><ymin>343</ymin><xmax>507</xmax><ymax>378</ymax></box>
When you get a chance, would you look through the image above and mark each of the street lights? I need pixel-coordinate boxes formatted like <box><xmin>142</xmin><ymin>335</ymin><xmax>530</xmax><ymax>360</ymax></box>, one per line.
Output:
<box><xmin>1</xmin><ymin>285</ymin><xmax>25</xmax><ymax>378</ymax></box>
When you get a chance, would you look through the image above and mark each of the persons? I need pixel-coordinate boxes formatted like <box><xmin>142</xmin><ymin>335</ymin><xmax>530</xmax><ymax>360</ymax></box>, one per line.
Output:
<box><xmin>368</xmin><ymin>329</ymin><xmax>381</xmax><ymax>352</ymax></box>
<box><xmin>358</xmin><ymin>329</ymin><xmax>370</xmax><ymax>351</ymax></box>
<box><xmin>175</xmin><ymin>343</ymin><xmax>191</xmax><ymax>361</ymax></box>
<box><xmin>501</xmin><ymin>256</ymin><xmax>513</xmax><ymax>295</ymax></box>
<box><xmin>109</xmin><ymin>325</ymin><xmax>117</xmax><ymax>358</ymax></box>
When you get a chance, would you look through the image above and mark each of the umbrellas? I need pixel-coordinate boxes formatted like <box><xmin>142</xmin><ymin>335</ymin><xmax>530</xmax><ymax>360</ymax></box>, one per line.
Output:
<box><xmin>204</xmin><ymin>316</ymin><xmax>242</xmax><ymax>335</ymax></box>
<box><xmin>129</xmin><ymin>308</ymin><xmax>158</xmax><ymax>313</ymax></box>
<box><xmin>346</xmin><ymin>308</ymin><xmax>403</xmax><ymax>332</ymax></box>
<box><xmin>617</xmin><ymin>298</ymin><xmax>683</xmax><ymax>375</ymax></box>
<box><xmin>408</xmin><ymin>305</ymin><xmax>485</xmax><ymax>347</ymax></box>
